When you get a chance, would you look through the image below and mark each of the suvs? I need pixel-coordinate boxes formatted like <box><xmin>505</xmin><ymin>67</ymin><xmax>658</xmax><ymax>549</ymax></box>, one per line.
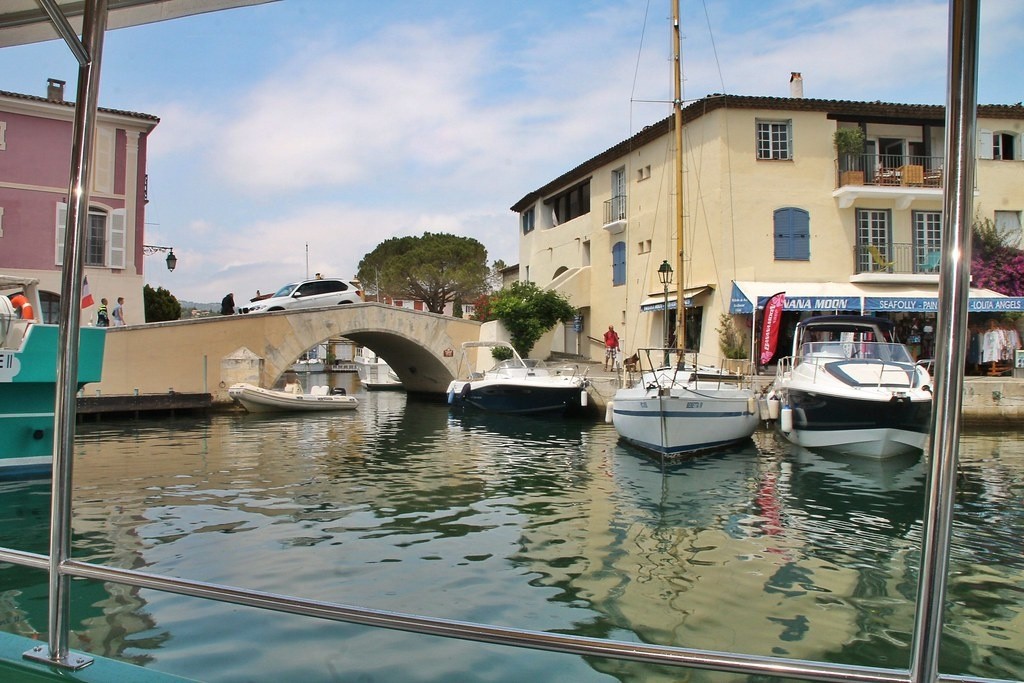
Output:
<box><xmin>236</xmin><ymin>278</ymin><xmax>363</xmax><ymax>315</ymax></box>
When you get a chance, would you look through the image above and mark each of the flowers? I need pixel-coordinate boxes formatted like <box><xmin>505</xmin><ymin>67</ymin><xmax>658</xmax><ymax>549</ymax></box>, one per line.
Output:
<box><xmin>970</xmin><ymin>202</ymin><xmax>1024</xmax><ymax>296</ymax></box>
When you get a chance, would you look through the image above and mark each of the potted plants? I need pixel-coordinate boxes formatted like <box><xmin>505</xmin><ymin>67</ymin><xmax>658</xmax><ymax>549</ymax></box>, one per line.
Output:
<box><xmin>715</xmin><ymin>312</ymin><xmax>752</xmax><ymax>374</ymax></box>
<box><xmin>831</xmin><ymin>126</ymin><xmax>866</xmax><ymax>186</ymax></box>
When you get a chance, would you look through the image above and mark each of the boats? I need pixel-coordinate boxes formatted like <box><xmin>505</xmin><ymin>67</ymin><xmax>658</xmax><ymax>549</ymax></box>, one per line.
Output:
<box><xmin>612</xmin><ymin>347</ymin><xmax>759</xmax><ymax>458</ymax></box>
<box><xmin>446</xmin><ymin>341</ymin><xmax>587</xmax><ymax>413</ymax></box>
<box><xmin>0</xmin><ymin>275</ymin><xmax>109</xmax><ymax>488</ymax></box>
<box><xmin>354</xmin><ymin>346</ymin><xmax>402</xmax><ymax>389</ymax></box>
<box><xmin>228</xmin><ymin>379</ymin><xmax>359</xmax><ymax>412</ymax></box>
<box><xmin>774</xmin><ymin>315</ymin><xmax>935</xmax><ymax>459</ymax></box>
<box><xmin>292</xmin><ymin>357</ymin><xmax>324</xmax><ymax>372</ymax></box>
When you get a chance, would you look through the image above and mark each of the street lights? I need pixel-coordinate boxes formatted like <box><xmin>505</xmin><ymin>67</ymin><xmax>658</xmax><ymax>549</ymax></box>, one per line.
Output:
<box><xmin>657</xmin><ymin>260</ymin><xmax>673</xmax><ymax>367</ymax></box>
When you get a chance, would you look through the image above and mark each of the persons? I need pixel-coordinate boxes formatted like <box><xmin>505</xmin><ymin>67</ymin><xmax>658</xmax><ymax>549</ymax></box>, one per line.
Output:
<box><xmin>220</xmin><ymin>293</ymin><xmax>235</xmax><ymax>316</ymax></box>
<box><xmin>603</xmin><ymin>325</ymin><xmax>620</xmax><ymax>372</ymax></box>
<box><xmin>113</xmin><ymin>297</ymin><xmax>126</xmax><ymax>326</ymax></box>
<box><xmin>315</xmin><ymin>273</ymin><xmax>323</xmax><ymax>279</ymax></box>
<box><xmin>97</xmin><ymin>298</ymin><xmax>109</xmax><ymax>326</ymax></box>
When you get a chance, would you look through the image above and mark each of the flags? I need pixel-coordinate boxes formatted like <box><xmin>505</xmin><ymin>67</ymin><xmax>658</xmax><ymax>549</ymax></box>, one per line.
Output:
<box><xmin>80</xmin><ymin>275</ymin><xmax>94</xmax><ymax>309</ymax></box>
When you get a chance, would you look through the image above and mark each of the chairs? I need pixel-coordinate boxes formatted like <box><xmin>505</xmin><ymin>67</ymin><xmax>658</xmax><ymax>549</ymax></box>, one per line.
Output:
<box><xmin>875</xmin><ymin>162</ymin><xmax>943</xmax><ymax>186</ymax></box>
<box><xmin>870</xmin><ymin>245</ymin><xmax>898</xmax><ymax>273</ymax></box>
<box><xmin>916</xmin><ymin>250</ymin><xmax>941</xmax><ymax>272</ymax></box>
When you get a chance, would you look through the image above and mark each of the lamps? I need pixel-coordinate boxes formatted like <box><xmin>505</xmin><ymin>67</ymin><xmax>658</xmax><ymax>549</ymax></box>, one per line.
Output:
<box><xmin>142</xmin><ymin>244</ymin><xmax>177</xmax><ymax>272</ymax></box>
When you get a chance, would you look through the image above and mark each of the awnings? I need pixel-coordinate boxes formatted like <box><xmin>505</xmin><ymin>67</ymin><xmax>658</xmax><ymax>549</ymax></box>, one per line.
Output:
<box><xmin>640</xmin><ymin>287</ymin><xmax>707</xmax><ymax>313</ymax></box>
<box><xmin>731</xmin><ymin>280</ymin><xmax>1024</xmax><ymax>332</ymax></box>
